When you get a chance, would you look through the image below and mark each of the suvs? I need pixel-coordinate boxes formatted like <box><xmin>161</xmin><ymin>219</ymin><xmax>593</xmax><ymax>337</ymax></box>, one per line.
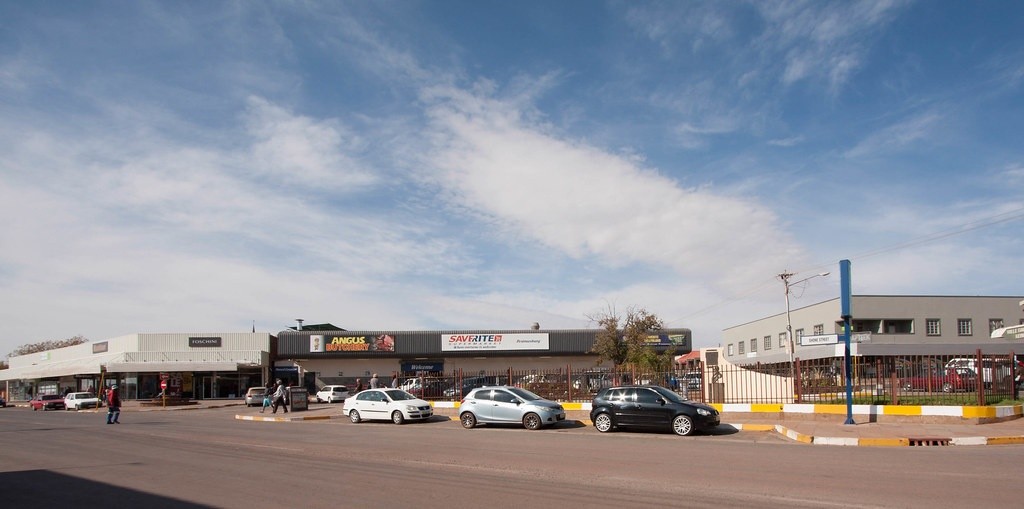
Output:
<box><xmin>396</xmin><ymin>377</ymin><xmax>439</xmax><ymax>392</ymax></box>
<box><xmin>590</xmin><ymin>383</ymin><xmax>721</xmax><ymax>436</ymax></box>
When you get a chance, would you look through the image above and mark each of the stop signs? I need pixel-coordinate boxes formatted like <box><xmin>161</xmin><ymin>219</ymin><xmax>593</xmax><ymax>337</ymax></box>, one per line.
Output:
<box><xmin>161</xmin><ymin>380</ymin><xmax>167</xmax><ymax>389</ymax></box>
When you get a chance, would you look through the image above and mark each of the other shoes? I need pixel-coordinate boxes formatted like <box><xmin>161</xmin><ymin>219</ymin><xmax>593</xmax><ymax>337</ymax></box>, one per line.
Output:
<box><xmin>107</xmin><ymin>422</ymin><xmax>113</xmax><ymax>424</ymax></box>
<box><xmin>114</xmin><ymin>419</ymin><xmax>120</xmax><ymax>424</ymax></box>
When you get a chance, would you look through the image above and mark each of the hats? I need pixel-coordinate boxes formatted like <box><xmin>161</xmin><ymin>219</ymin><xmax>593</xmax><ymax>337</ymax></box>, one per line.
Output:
<box><xmin>112</xmin><ymin>384</ymin><xmax>118</xmax><ymax>389</ymax></box>
<box><xmin>373</xmin><ymin>374</ymin><xmax>378</xmax><ymax>378</ymax></box>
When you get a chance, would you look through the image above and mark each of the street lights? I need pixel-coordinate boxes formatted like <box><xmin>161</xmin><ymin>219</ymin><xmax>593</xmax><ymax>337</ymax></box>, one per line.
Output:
<box><xmin>785</xmin><ymin>272</ymin><xmax>832</xmax><ymax>377</ymax></box>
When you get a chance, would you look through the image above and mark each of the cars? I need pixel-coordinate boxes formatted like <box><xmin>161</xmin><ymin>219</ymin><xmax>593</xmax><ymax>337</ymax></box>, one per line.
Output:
<box><xmin>913</xmin><ymin>357</ymin><xmax>1024</xmax><ymax>391</ymax></box>
<box><xmin>859</xmin><ymin>357</ymin><xmax>916</xmax><ymax>378</ymax></box>
<box><xmin>64</xmin><ymin>391</ymin><xmax>102</xmax><ymax>411</ymax></box>
<box><xmin>898</xmin><ymin>367</ymin><xmax>978</xmax><ymax>393</ymax></box>
<box><xmin>268</xmin><ymin>386</ymin><xmax>289</xmax><ymax>406</ymax></box>
<box><xmin>29</xmin><ymin>393</ymin><xmax>65</xmax><ymax>411</ymax></box>
<box><xmin>404</xmin><ymin>381</ymin><xmax>454</xmax><ymax>401</ymax></box>
<box><xmin>458</xmin><ymin>386</ymin><xmax>566</xmax><ymax>430</ymax></box>
<box><xmin>244</xmin><ymin>386</ymin><xmax>267</xmax><ymax>407</ymax></box>
<box><xmin>443</xmin><ymin>371</ymin><xmax>680</xmax><ymax>401</ymax></box>
<box><xmin>316</xmin><ymin>385</ymin><xmax>351</xmax><ymax>404</ymax></box>
<box><xmin>0</xmin><ymin>396</ymin><xmax>6</xmax><ymax>407</ymax></box>
<box><xmin>679</xmin><ymin>371</ymin><xmax>702</xmax><ymax>392</ymax></box>
<box><xmin>343</xmin><ymin>387</ymin><xmax>433</xmax><ymax>425</ymax></box>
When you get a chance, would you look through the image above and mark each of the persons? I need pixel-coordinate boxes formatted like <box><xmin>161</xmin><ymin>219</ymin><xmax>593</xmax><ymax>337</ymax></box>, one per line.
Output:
<box><xmin>392</xmin><ymin>375</ymin><xmax>397</xmax><ymax>389</ymax></box>
<box><xmin>270</xmin><ymin>380</ymin><xmax>288</xmax><ymax>414</ymax></box>
<box><xmin>106</xmin><ymin>385</ymin><xmax>122</xmax><ymax>425</ymax></box>
<box><xmin>85</xmin><ymin>385</ymin><xmax>96</xmax><ymax>397</ymax></box>
<box><xmin>354</xmin><ymin>378</ymin><xmax>362</xmax><ymax>393</ymax></box>
<box><xmin>64</xmin><ymin>385</ymin><xmax>73</xmax><ymax>397</ymax></box>
<box><xmin>102</xmin><ymin>386</ymin><xmax>107</xmax><ymax>403</ymax></box>
<box><xmin>259</xmin><ymin>382</ymin><xmax>277</xmax><ymax>413</ymax></box>
<box><xmin>370</xmin><ymin>373</ymin><xmax>380</xmax><ymax>389</ymax></box>
